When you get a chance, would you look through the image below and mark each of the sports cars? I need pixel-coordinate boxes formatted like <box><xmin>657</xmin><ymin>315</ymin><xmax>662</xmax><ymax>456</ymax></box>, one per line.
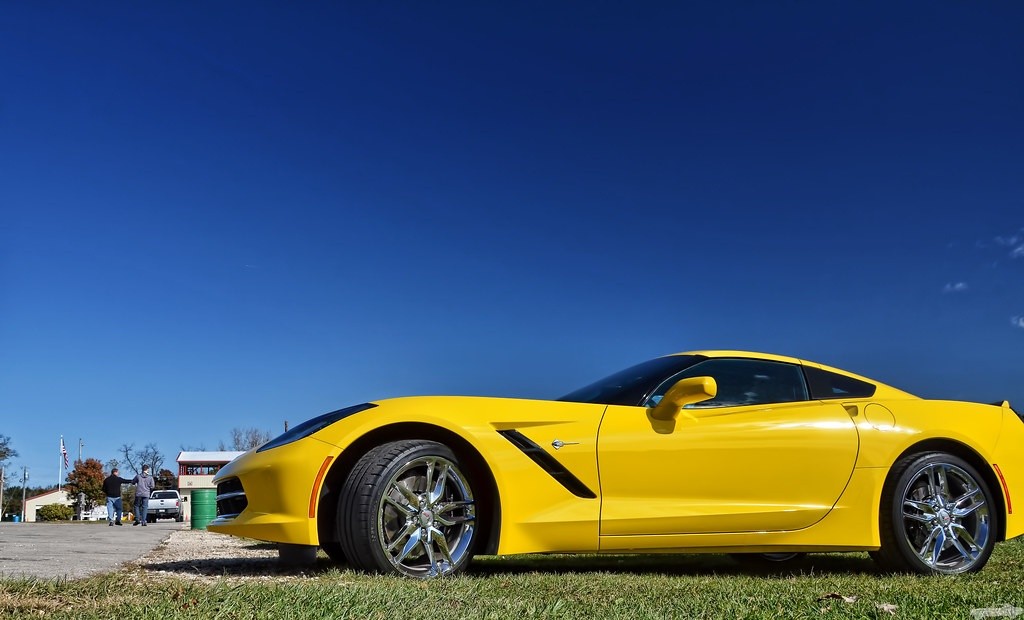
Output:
<box><xmin>206</xmin><ymin>349</ymin><xmax>1024</xmax><ymax>577</ymax></box>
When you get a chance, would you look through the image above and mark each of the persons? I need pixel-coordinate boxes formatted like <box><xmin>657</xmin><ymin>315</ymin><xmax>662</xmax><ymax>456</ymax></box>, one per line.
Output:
<box><xmin>102</xmin><ymin>469</ymin><xmax>129</xmax><ymax>526</ymax></box>
<box><xmin>131</xmin><ymin>464</ymin><xmax>154</xmax><ymax>526</ymax></box>
<box><xmin>189</xmin><ymin>468</ymin><xmax>198</xmax><ymax>475</ymax></box>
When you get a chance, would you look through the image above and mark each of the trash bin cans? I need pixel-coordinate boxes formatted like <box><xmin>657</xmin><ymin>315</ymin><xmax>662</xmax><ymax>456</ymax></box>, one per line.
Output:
<box><xmin>13</xmin><ymin>516</ymin><xmax>18</xmax><ymax>523</ymax></box>
<box><xmin>190</xmin><ymin>488</ymin><xmax>217</xmax><ymax>530</ymax></box>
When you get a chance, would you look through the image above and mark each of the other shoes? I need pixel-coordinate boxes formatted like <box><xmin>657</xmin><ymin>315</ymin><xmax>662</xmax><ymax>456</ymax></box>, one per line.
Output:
<box><xmin>109</xmin><ymin>522</ymin><xmax>114</xmax><ymax>526</ymax></box>
<box><xmin>115</xmin><ymin>521</ymin><xmax>122</xmax><ymax>525</ymax></box>
<box><xmin>133</xmin><ymin>519</ymin><xmax>140</xmax><ymax>525</ymax></box>
<box><xmin>142</xmin><ymin>522</ymin><xmax>147</xmax><ymax>526</ymax></box>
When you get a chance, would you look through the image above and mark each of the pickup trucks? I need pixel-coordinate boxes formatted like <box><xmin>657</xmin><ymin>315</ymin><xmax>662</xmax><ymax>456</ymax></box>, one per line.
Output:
<box><xmin>147</xmin><ymin>490</ymin><xmax>187</xmax><ymax>523</ymax></box>
